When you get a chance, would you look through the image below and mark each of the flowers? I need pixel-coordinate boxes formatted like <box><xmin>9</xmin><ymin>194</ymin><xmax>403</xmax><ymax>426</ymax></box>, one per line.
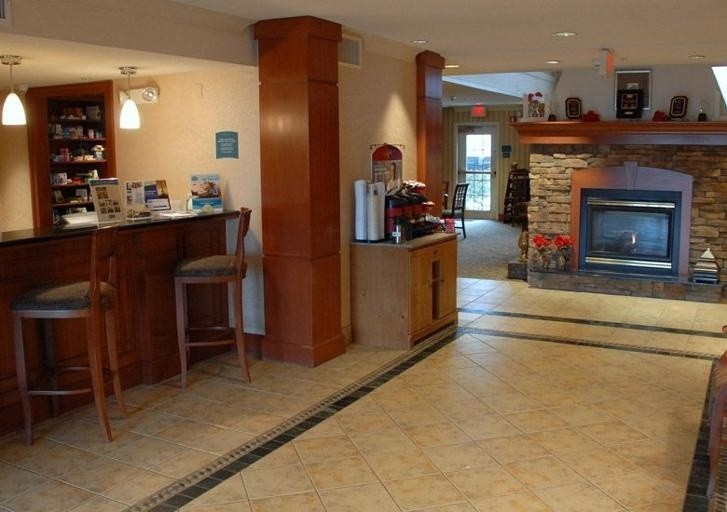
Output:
<box><xmin>533</xmin><ymin>233</ymin><xmax>576</xmax><ymax>257</ymax></box>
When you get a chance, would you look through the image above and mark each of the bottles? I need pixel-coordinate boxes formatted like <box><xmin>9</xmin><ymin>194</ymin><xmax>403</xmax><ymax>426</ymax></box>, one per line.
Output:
<box><xmin>391</xmin><ymin>217</ymin><xmax>406</xmax><ymax>245</ymax></box>
<box><xmin>94</xmin><ymin>148</ymin><xmax>103</xmax><ymax>161</ymax></box>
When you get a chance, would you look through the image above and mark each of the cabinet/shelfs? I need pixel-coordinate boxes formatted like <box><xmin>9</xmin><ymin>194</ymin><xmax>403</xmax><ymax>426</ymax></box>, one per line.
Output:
<box><xmin>45</xmin><ymin>98</ymin><xmax>110</xmax><ymax>210</ymax></box>
<box><xmin>352</xmin><ymin>233</ymin><xmax>459</xmax><ymax>351</ymax></box>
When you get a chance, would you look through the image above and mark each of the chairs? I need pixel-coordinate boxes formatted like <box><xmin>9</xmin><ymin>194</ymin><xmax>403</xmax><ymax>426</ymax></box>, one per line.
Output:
<box><xmin>164</xmin><ymin>204</ymin><xmax>256</xmax><ymax>393</ymax></box>
<box><xmin>439</xmin><ymin>182</ymin><xmax>469</xmax><ymax>239</ymax></box>
<box><xmin>9</xmin><ymin>221</ymin><xmax>129</xmax><ymax>446</ymax></box>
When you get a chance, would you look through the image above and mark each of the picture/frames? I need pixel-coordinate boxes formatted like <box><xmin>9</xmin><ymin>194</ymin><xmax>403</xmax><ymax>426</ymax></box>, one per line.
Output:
<box><xmin>613</xmin><ymin>69</ymin><xmax>652</xmax><ymax>119</ymax></box>
<box><xmin>565</xmin><ymin>96</ymin><xmax>581</xmax><ymax>119</ymax></box>
<box><xmin>669</xmin><ymin>96</ymin><xmax>688</xmax><ymax>117</ymax></box>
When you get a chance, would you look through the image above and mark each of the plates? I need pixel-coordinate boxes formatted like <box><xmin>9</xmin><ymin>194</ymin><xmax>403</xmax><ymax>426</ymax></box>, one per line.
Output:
<box><xmin>124</xmin><ymin>215</ymin><xmax>155</xmax><ymax>221</ymax></box>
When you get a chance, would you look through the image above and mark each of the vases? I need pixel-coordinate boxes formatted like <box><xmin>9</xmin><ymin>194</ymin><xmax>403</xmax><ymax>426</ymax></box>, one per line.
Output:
<box><xmin>541</xmin><ymin>254</ymin><xmax>568</xmax><ymax>272</ymax></box>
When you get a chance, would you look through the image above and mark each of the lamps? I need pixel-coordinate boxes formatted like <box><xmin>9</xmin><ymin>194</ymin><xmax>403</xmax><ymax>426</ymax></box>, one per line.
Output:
<box><xmin>118</xmin><ymin>66</ymin><xmax>141</xmax><ymax>130</ymax></box>
<box><xmin>0</xmin><ymin>55</ymin><xmax>28</xmax><ymax>126</ymax></box>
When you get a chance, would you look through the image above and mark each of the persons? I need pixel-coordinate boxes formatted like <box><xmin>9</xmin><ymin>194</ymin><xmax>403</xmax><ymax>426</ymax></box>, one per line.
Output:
<box><xmin>387</xmin><ymin>161</ymin><xmax>401</xmax><ymax>193</ymax></box>
